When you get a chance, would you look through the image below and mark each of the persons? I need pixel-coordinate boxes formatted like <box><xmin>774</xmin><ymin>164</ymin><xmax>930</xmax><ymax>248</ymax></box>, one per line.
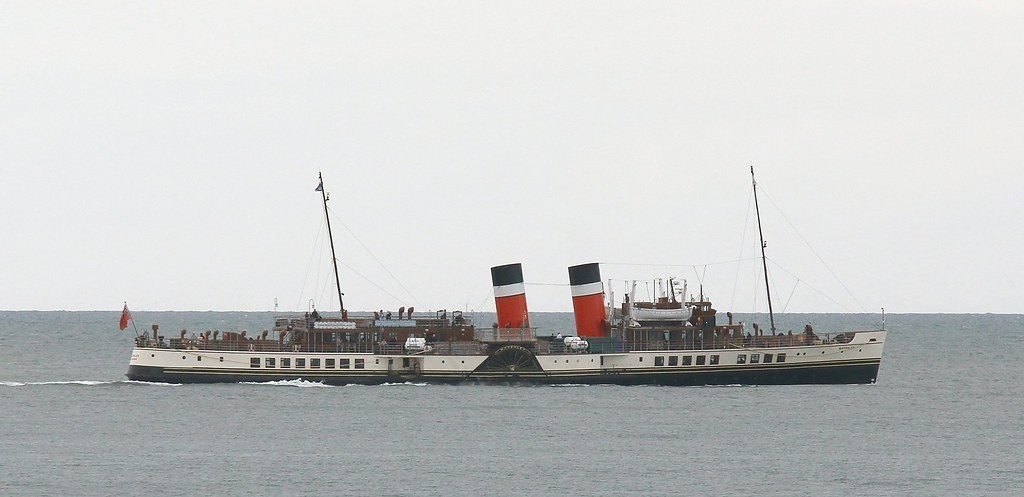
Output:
<box><xmin>191</xmin><ymin>332</ymin><xmax>205</xmax><ymax>339</ymax></box>
<box><xmin>304</xmin><ymin>309</ymin><xmax>318</xmax><ymax>317</ymax></box>
<box><xmin>805</xmin><ymin>322</ymin><xmax>813</xmax><ymax>332</ymax></box>
<box><xmin>381</xmin><ymin>339</ymin><xmax>387</xmax><ymax>354</ymax></box>
<box><xmin>373</xmin><ymin>309</ymin><xmax>384</xmax><ymax>317</ymax></box>
<box><xmin>550</xmin><ymin>332</ymin><xmax>562</xmax><ymax>341</ymax></box>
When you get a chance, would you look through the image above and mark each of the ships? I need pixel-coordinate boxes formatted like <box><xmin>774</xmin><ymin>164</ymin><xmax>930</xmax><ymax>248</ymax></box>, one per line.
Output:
<box><xmin>119</xmin><ymin>165</ymin><xmax>887</xmax><ymax>388</ymax></box>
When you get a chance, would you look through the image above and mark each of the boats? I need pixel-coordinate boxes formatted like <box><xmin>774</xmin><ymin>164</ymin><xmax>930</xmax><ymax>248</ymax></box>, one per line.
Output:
<box><xmin>630</xmin><ymin>306</ymin><xmax>694</xmax><ymax>322</ymax></box>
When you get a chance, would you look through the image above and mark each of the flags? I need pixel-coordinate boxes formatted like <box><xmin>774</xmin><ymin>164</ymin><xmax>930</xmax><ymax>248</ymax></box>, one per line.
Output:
<box><xmin>315</xmin><ymin>183</ymin><xmax>322</xmax><ymax>191</ymax></box>
<box><xmin>120</xmin><ymin>304</ymin><xmax>131</xmax><ymax>331</ymax></box>
